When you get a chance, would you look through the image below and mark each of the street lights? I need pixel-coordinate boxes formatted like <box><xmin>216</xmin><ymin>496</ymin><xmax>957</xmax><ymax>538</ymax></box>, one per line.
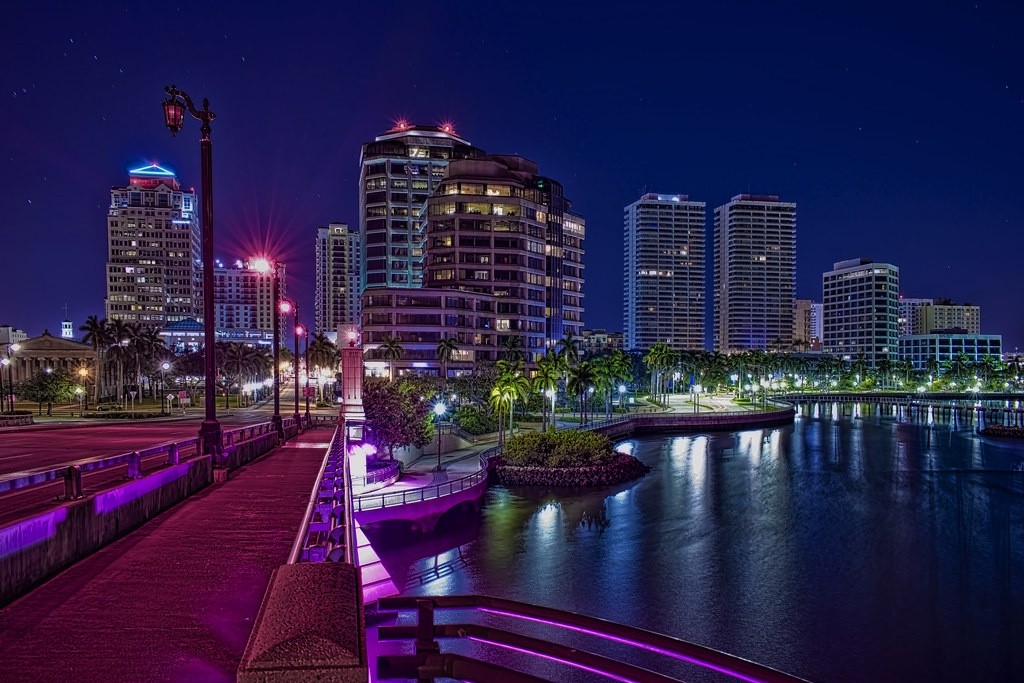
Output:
<box><xmin>546</xmin><ymin>389</ymin><xmax>554</xmax><ymax>430</ymax></box>
<box><xmin>1</xmin><ymin>343</ymin><xmax>20</xmax><ymax>412</ymax></box>
<box><xmin>589</xmin><ymin>387</ymin><xmax>596</xmax><ymax>426</ymax></box>
<box><xmin>162</xmin><ymin>85</ymin><xmax>220</xmax><ymax>437</ymax></box>
<box><xmin>78</xmin><ymin>368</ymin><xmax>89</xmax><ymax>409</ymax></box>
<box><xmin>745</xmin><ymin>379</ymin><xmax>786</xmax><ymax>412</ymax></box>
<box><xmin>695</xmin><ymin>384</ymin><xmax>702</xmax><ymax>414</ymax></box>
<box><xmin>618</xmin><ymin>385</ymin><xmax>626</xmax><ymax>421</ymax></box>
<box><xmin>252</xmin><ymin>259</ymin><xmax>284</xmax><ymax>422</ymax></box>
<box><xmin>281</xmin><ymin>298</ymin><xmax>300</xmax><ymax>418</ymax></box>
<box><xmin>433</xmin><ymin>403</ymin><xmax>445</xmax><ymax>473</ymax></box>
<box><xmin>294</xmin><ymin>322</ymin><xmax>311</xmax><ymax>417</ymax></box>
